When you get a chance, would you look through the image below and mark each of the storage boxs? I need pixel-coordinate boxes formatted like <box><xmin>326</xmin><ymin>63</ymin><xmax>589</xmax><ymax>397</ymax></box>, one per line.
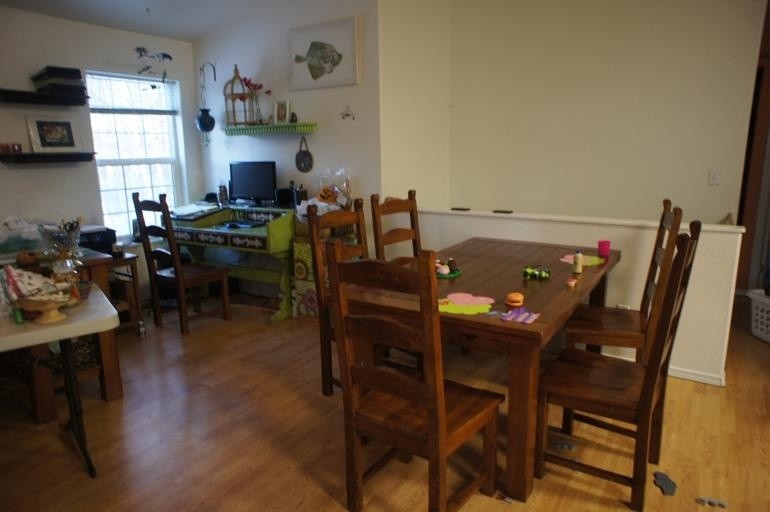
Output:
<box><xmin>292</xmin><ymin>238</ymin><xmax>359</xmax><ymax>320</ymax></box>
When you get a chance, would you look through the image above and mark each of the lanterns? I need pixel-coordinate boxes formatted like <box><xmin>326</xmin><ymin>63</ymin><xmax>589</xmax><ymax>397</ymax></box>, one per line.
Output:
<box><xmin>318</xmin><ymin>185</ymin><xmax>336</xmax><ymax>203</ymax></box>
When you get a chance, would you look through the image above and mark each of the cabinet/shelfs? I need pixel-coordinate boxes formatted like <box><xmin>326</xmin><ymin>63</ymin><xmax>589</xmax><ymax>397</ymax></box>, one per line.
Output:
<box><xmin>0</xmin><ymin>87</ymin><xmax>95</xmax><ymax>165</ymax></box>
<box><xmin>160</xmin><ymin>197</ymin><xmax>295</xmax><ymax>324</ymax></box>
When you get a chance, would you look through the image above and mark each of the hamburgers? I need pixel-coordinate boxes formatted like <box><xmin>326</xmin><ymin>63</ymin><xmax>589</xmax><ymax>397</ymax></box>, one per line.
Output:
<box><xmin>505</xmin><ymin>292</ymin><xmax>524</xmax><ymax>307</ymax></box>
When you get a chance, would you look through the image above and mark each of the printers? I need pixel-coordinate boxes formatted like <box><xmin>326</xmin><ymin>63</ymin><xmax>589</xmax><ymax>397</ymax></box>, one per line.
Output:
<box><xmin>77</xmin><ymin>223</ymin><xmax>116</xmax><ymax>253</ymax></box>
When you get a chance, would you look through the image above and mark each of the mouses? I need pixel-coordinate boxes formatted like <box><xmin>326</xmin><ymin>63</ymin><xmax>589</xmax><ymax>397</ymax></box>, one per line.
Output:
<box><xmin>228</xmin><ymin>224</ymin><xmax>239</xmax><ymax>228</ymax></box>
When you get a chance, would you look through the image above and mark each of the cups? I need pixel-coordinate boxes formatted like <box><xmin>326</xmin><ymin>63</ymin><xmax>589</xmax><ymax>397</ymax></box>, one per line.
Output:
<box><xmin>598</xmin><ymin>240</ymin><xmax>610</xmax><ymax>259</ymax></box>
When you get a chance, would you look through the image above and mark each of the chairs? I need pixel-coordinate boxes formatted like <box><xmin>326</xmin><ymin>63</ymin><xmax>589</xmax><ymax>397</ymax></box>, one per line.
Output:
<box><xmin>307</xmin><ymin>199</ymin><xmax>369</xmax><ymax>397</ymax></box>
<box><xmin>132</xmin><ymin>191</ymin><xmax>232</xmax><ymax>334</ymax></box>
<box><xmin>370</xmin><ymin>189</ymin><xmax>423</xmax><ymax>379</ymax></box>
<box><xmin>535</xmin><ymin>219</ymin><xmax>702</xmax><ymax>510</ymax></box>
<box><xmin>563</xmin><ymin>200</ymin><xmax>684</xmax><ymax>362</ymax></box>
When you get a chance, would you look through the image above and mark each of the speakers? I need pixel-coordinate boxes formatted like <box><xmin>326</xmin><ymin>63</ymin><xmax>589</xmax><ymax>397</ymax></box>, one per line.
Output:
<box><xmin>275</xmin><ymin>188</ymin><xmax>291</xmax><ymax>204</ymax></box>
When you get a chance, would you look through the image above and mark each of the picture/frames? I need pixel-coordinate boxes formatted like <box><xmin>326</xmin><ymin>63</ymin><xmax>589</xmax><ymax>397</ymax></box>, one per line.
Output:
<box><xmin>25</xmin><ymin>113</ymin><xmax>85</xmax><ymax>152</ymax></box>
<box><xmin>287</xmin><ymin>15</ymin><xmax>360</xmax><ymax>92</ymax></box>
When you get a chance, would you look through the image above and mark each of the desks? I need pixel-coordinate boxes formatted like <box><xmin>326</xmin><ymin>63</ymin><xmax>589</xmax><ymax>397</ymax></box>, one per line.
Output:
<box><xmin>89</xmin><ymin>251</ymin><xmax>142</xmax><ymax>332</ymax></box>
<box><xmin>329</xmin><ymin>235</ymin><xmax>622</xmax><ymax>504</ymax></box>
<box><xmin>0</xmin><ymin>241</ymin><xmax>126</xmax><ymax>478</ymax></box>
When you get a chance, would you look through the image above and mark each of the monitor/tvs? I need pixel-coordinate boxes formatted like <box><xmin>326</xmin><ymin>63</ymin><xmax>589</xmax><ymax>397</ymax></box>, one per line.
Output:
<box><xmin>228</xmin><ymin>160</ymin><xmax>277</xmax><ymax>207</ymax></box>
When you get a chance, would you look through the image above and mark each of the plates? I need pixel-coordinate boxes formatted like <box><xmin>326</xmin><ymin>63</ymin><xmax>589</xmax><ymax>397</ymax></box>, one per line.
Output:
<box><xmin>437</xmin><ymin>269</ymin><xmax>462</xmax><ymax>279</ymax></box>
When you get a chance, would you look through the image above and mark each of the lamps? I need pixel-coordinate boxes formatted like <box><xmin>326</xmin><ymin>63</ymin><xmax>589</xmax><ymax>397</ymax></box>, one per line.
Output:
<box><xmin>193</xmin><ymin>61</ymin><xmax>216</xmax><ymax>134</ymax></box>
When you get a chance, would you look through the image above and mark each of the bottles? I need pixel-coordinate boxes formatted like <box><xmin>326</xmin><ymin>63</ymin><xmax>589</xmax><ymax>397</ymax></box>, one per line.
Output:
<box><xmin>195</xmin><ymin>108</ymin><xmax>215</xmax><ymax>131</ymax></box>
<box><xmin>435</xmin><ymin>258</ymin><xmax>457</xmax><ymax>275</ymax></box>
<box><xmin>573</xmin><ymin>250</ymin><xmax>583</xmax><ymax>273</ymax></box>
<box><xmin>217</xmin><ymin>179</ymin><xmax>228</xmax><ymax>204</ymax></box>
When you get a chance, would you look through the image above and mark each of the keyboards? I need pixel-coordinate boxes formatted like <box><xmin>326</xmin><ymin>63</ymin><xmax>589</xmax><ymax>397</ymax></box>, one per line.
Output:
<box><xmin>224</xmin><ymin>220</ymin><xmax>264</xmax><ymax>228</ymax></box>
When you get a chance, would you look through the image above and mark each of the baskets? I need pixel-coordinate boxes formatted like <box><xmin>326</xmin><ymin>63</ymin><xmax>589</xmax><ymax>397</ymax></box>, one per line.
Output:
<box><xmin>745</xmin><ymin>287</ymin><xmax>770</xmax><ymax>342</ymax></box>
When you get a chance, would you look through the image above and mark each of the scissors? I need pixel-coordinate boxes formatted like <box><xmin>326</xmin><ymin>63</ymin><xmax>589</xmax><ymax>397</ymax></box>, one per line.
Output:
<box><xmin>64</xmin><ymin>221</ymin><xmax>78</xmax><ymax>235</ymax></box>
